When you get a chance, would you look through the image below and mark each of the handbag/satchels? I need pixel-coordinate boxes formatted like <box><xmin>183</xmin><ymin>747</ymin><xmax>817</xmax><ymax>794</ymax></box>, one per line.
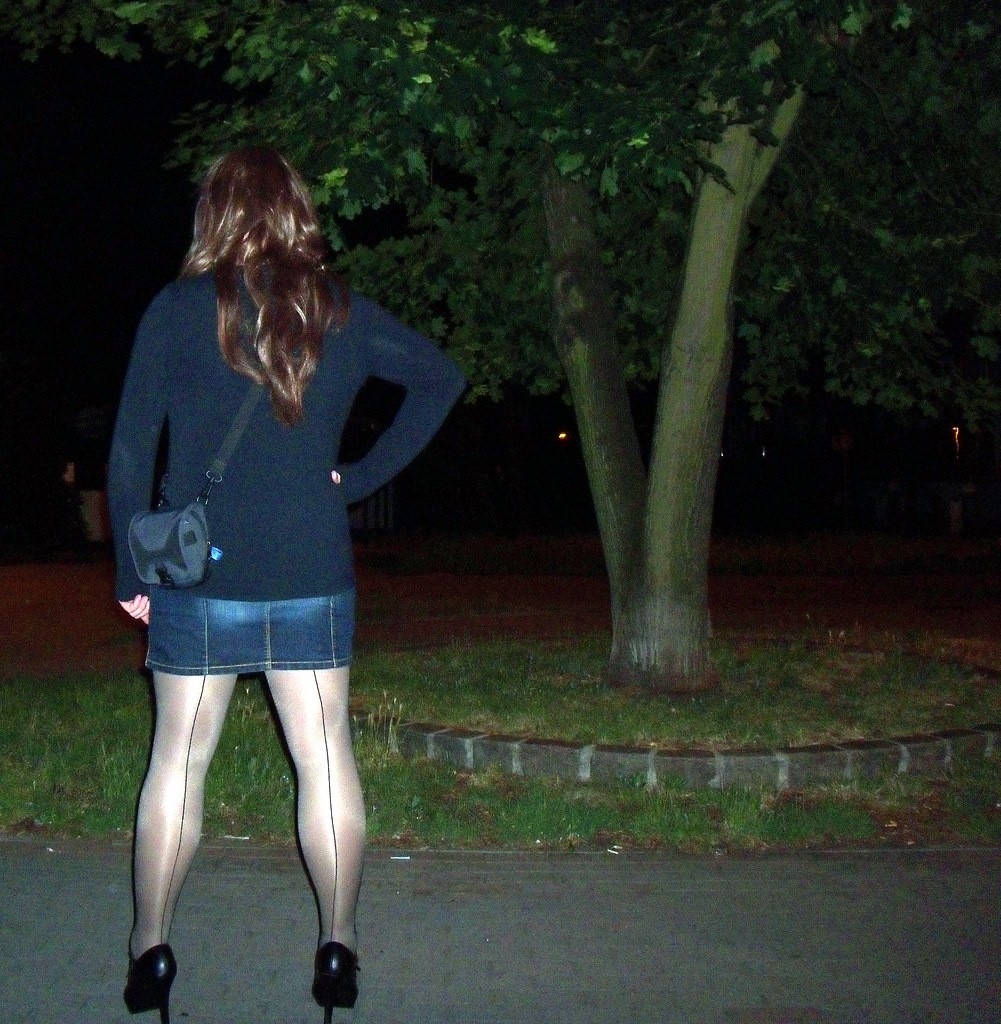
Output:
<box><xmin>127</xmin><ymin>496</ymin><xmax>214</xmax><ymax>590</ymax></box>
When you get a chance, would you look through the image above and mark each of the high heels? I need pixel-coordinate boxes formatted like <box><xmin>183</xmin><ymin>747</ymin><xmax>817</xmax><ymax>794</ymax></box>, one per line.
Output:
<box><xmin>124</xmin><ymin>942</ymin><xmax>178</xmax><ymax>1022</ymax></box>
<box><xmin>314</xmin><ymin>941</ymin><xmax>361</xmax><ymax>1023</ymax></box>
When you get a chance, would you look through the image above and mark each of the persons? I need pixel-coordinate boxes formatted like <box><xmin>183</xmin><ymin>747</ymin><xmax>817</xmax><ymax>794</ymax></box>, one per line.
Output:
<box><xmin>105</xmin><ymin>143</ymin><xmax>467</xmax><ymax>1024</ymax></box>
